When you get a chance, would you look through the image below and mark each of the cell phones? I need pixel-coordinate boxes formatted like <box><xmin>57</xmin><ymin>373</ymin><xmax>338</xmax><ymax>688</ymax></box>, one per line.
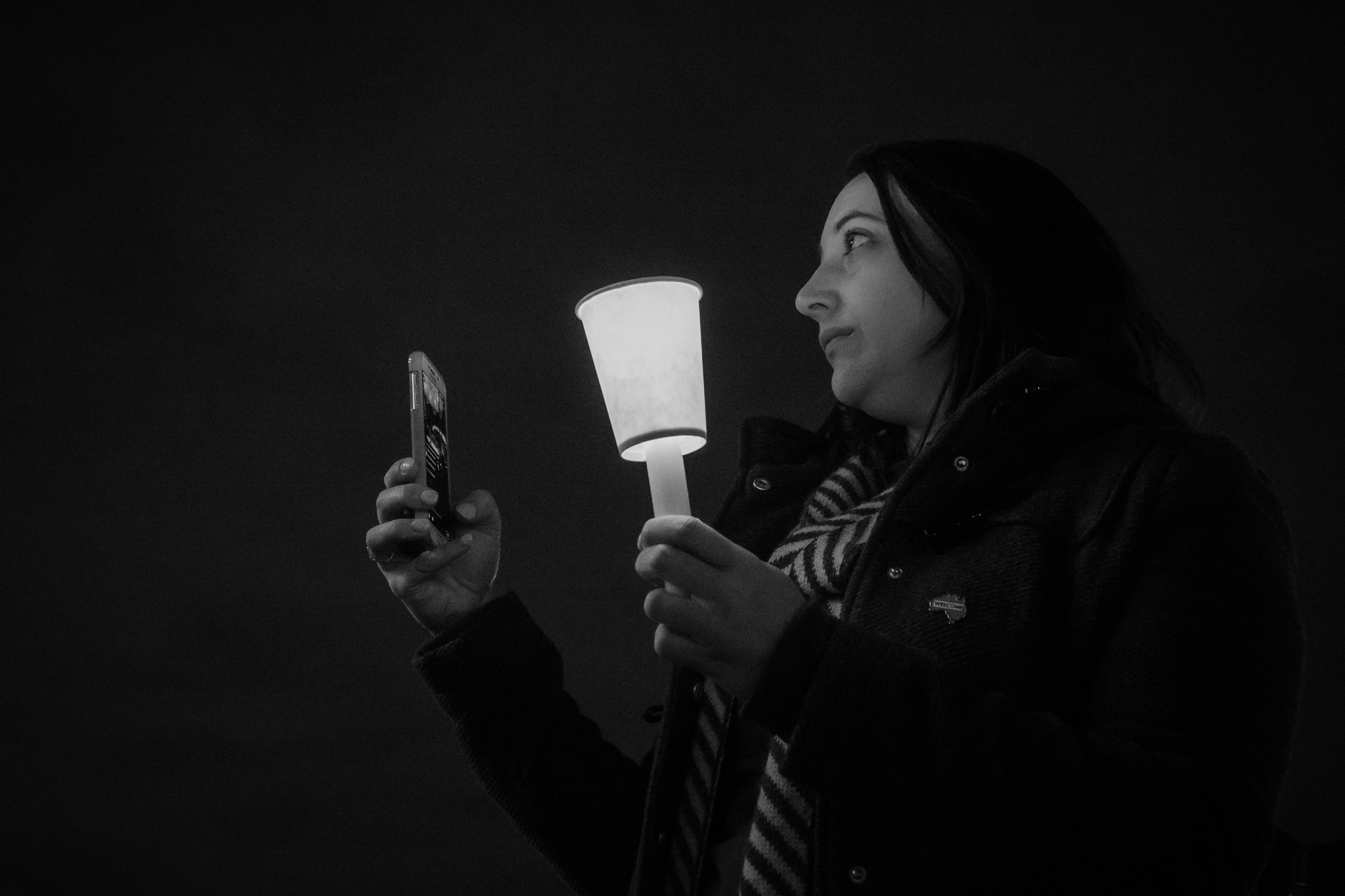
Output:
<box><xmin>408</xmin><ymin>350</ymin><xmax>454</xmax><ymax>549</ymax></box>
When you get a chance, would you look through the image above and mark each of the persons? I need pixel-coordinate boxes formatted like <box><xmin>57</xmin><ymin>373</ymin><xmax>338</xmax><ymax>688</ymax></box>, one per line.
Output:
<box><xmin>364</xmin><ymin>139</ymin><xmax>1290</xmax><ymax>896</ymax></box>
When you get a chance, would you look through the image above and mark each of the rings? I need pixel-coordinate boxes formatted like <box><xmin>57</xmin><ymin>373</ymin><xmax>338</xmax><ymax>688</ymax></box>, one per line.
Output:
<box><xmin>366</xmin><ymin>543</ymin><xmax>396</xmax><ymax>563</ymax></box>
